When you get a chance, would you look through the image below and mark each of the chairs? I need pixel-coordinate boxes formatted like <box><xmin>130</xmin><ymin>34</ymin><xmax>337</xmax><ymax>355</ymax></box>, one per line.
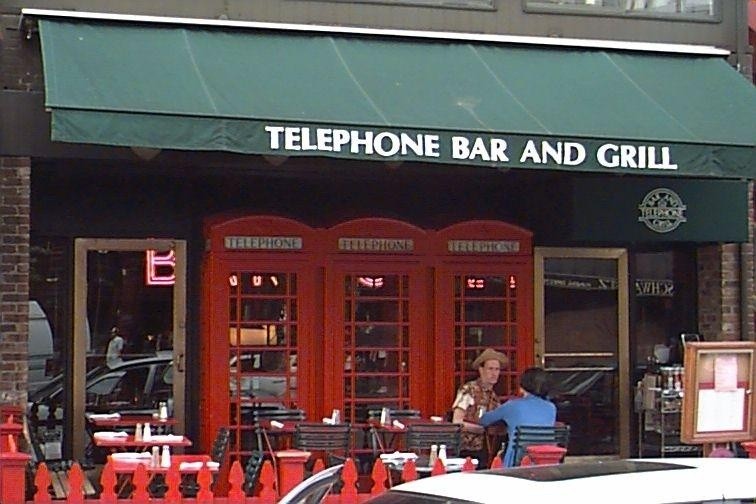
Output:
<box><xmin>637</xmin><ymin>380</ymin><xmax>703</xmax><ymax>457</ymax></box>
<box><xmin>27</xmin><ymin>409</ymin><xmax>570</xmax><ymax>500</ymax></box>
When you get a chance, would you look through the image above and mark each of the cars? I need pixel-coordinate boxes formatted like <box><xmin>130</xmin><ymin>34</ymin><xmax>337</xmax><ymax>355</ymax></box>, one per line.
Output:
<box><xmin>272</xmin><ymin>455</ymin><xmax>756</xmax><ymax>504</ymax></box>
<box><xmin>25</xmin><ymin>352</ymin><xmax>299</xmax><ymax>441</ymax></box>
<box><xmin>543</xmin><ymin>363</ymin><xmax>650</xmax><ymax>446</ymax></box>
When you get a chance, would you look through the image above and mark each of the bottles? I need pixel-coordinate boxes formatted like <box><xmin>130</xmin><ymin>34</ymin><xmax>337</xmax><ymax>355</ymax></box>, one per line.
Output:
<box><xmin>659</xmin><ymin>365</ymin><xmax>685</xmax><ymax>390</ymax></box>
<box><xmin>142</xmin><ymin>422</ymin><xmax>152</xmax><ymax>442</ymax></box>
<box><xmin>134</xmin><ymin>422</ymin><xmax>142</xmax><ymax>441</ymax></box>
<box><xmin>439</xmin><ymin>444</ymin><xmax>447</xmax><ymax>465</ymax></box>
<box><xmin>162</xmin><ymin>445</ymin><xmax>172</xmax><ymax>467</ymax></box>
<box><xmin>158</xmin><ymin>400</ymin><xmax>167</xmax><ymax>418</ymax></box>
<box><xmin>429</xmin><ymin>444</ymin><xmax>438</xmax><ymax>465</ymax></box>
<box><xmin>151</xmin><ymin>445</ymin><xmax>160</xmax><ymax>467</ymax></box>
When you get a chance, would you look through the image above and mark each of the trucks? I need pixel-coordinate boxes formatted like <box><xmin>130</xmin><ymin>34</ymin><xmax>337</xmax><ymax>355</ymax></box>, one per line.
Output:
<box><xmin>28</xmin><ymin>300</ymin><xmax>58</xmax><ymax>390</ymax></box>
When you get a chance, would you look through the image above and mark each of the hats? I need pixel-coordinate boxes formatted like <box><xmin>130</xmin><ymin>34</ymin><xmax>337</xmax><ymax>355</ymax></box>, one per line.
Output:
<box><xmin>471</xmin><ymin>347</ymin><xmax>509</xmax><ymax>370</ymax></box>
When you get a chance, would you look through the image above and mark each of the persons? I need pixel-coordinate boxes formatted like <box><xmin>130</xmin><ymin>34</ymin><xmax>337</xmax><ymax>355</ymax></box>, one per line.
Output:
<box><xmin>478</xmin><ymin>367</ymin><xmax>562</xmax><ymax>467</ymax></box>
<box><xmin>451</xmin><ymin>347</ymin><xmax>510</xmax><ymax>468</ymax></box>
<box><xmin>104</xmin><ymin>325</ymin><xmax>124</xmax><ymax>361</ymax></box>
